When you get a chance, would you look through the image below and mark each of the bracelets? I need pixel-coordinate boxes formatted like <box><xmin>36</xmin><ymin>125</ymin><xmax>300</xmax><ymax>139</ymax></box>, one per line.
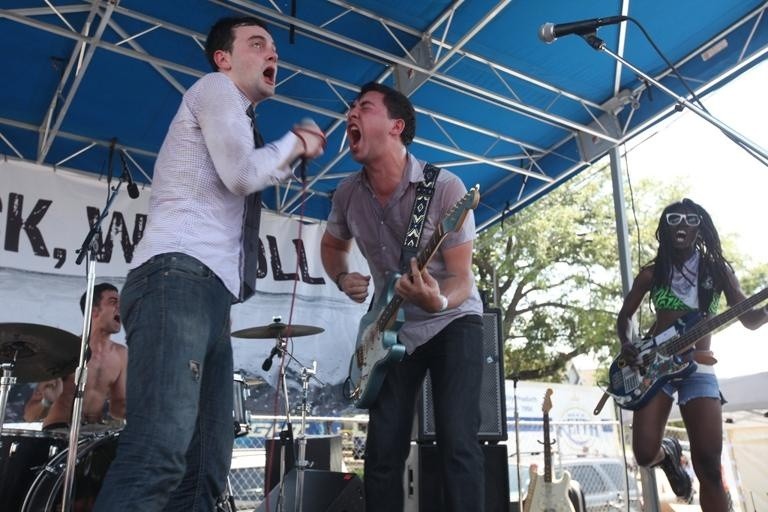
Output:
<box><xmin>41</xmin><ymin>397</ymin><xmax>54</xmax><ymax>407</ymax></box>
<box><xmin>336</xmin><ymin>271</ymin><xmax>348</xmax><ymax>292</ymax></box>
<box><xmin>438</xmin><ymin>295</ymin><xmax>448</xmax><ymax>312</ymax></box>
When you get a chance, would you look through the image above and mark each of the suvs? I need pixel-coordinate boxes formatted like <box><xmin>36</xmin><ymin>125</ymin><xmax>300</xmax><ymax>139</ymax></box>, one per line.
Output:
<box><xmin>504</xmin><ymin>455</ymin><xmax>639</xmax><ymax>512</ymax></box>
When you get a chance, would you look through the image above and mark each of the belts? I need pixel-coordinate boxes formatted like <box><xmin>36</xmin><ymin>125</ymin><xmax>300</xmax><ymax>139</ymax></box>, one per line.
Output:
<box><xmin>686</xmin><ymin>351</ymin><xmax>719</xmax><ymax>365</ymax></box>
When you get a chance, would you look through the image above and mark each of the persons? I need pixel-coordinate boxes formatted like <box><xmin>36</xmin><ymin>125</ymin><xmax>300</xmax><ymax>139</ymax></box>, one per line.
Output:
<box><xmin>24</xmin><ymin>282</ymin><xmax>128</xmax><ymax>429</ymax></box>
<box><xmin>94</xmin><ymin>17</ymin><xmax>326</xmax><ymax>512</ymax></box>
<box><xmin>321</xmin><ymin>81</ymin><xmax>485</xmax><ymax>512</ymax></box>
<box><xmin>616</xmin><ymin>198</ymin><xmax>768</xmax><ymax>511</ymax></box>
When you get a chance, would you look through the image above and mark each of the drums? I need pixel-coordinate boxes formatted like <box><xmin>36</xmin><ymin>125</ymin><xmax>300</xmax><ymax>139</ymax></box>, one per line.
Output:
<box><xmin>233</xmin><ymin>372</ymin><xmax>251</xmax><ymax>437</ymax></box>
<box><xmin>0</xmin><ymin>429</ymin><xmax>67</xmax><ymax>511</ymax></box>
<box><xmin>21</xmin><ymin>430</ymin><xmax>122</xmax><ymax>511</ymax></box>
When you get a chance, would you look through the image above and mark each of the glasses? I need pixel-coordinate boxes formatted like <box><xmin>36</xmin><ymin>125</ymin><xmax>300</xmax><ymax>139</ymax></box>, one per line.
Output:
<box><xmin>665</xmin><ymin>212</ymin><xmax>704</xmax><ymax>227</ymax></box>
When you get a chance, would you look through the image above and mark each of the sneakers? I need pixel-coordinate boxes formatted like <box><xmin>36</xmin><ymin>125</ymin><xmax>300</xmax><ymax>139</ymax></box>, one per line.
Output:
<box><xmin>660</xmin><ymin>436</ymin><xmax>691</xmax><ymax>500</ymax></box>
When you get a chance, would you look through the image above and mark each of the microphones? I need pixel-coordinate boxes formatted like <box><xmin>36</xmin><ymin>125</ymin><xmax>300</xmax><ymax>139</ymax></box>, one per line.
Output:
<box><xmin>298</xmin><ymin>117</ymin><xmax>315</xmax><ymax>185</ymax></box>
<box><xmin>118</xmin><ymin>154</ymin><xmax>140</xmax><ymax>200</ymax></box>
<box><xmin>537</xmin><ymin>13</ymin><xmax>627</xmax><ymax>45</ymax></box>
<box><xmin>260</xmin><ymin>346</ymin><xmax>278</xmax><ymax>371</ymax></box>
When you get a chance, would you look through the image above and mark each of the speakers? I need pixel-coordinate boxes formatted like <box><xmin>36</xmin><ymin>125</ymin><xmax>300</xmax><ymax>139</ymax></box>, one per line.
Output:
<box><xmin>412</xmin><ymin>308</ymin><xmax>507</xmax><ymax>445</ymax></box>
<box><xmin>254</xmin><ymin>470</ymin><xmax>366</xmax><ymax>512</ymax></box>
<box><xmin>403</xmin><ymin>445</ymin><xmax>510</xmax><ymax>512</ymax></box>
<box><xmin>265</xmin><ymin>433</ymin><xmax>344</xmax><ymax>494</ymax></box>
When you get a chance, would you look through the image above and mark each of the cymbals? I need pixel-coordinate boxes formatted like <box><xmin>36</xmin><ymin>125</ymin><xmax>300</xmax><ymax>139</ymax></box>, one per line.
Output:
<box><xmin>1</xmin><ymin>323</ymin><xmax>92</xmax><ymax>384</ymax></box>
<box><xmin>230</xmin><ymin>323</ymin><xmax>325</xmax><ymax>338</ymax></box>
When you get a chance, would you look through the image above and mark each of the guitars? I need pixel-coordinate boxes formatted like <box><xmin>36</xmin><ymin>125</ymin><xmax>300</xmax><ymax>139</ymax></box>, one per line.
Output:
<box><xmin>522</xmin><ymin>389</ymin><xmax>577</xmax><ymax>512</ymax></box>
<box><xmin>609</xmin><ymin>288</ymin><xmax>767</xmax><ymax>410</ymax></box>
<box><xmin>349</xmin><ymin>183</ymin><xmax>480</xmax><ymax>409</ymax></box>
